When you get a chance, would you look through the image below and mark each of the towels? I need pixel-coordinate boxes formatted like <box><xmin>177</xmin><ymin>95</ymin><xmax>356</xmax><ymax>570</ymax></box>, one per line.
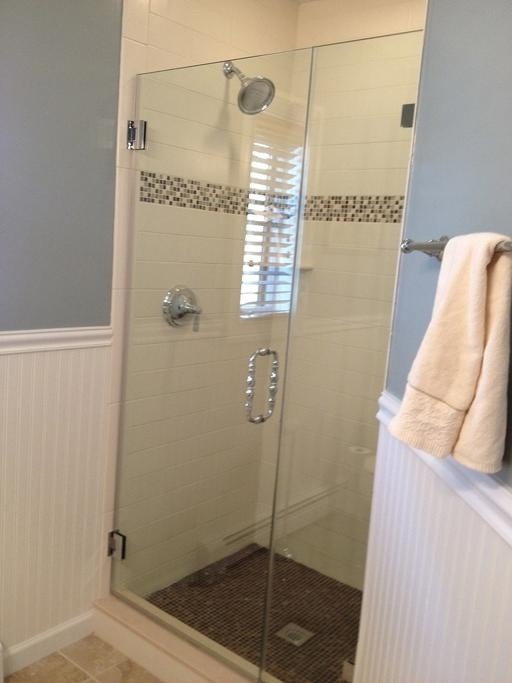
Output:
<box><xmin>386</xmin><ymin>232</ymin><xmax>512</xmax><ymax>475</ymax></box>
